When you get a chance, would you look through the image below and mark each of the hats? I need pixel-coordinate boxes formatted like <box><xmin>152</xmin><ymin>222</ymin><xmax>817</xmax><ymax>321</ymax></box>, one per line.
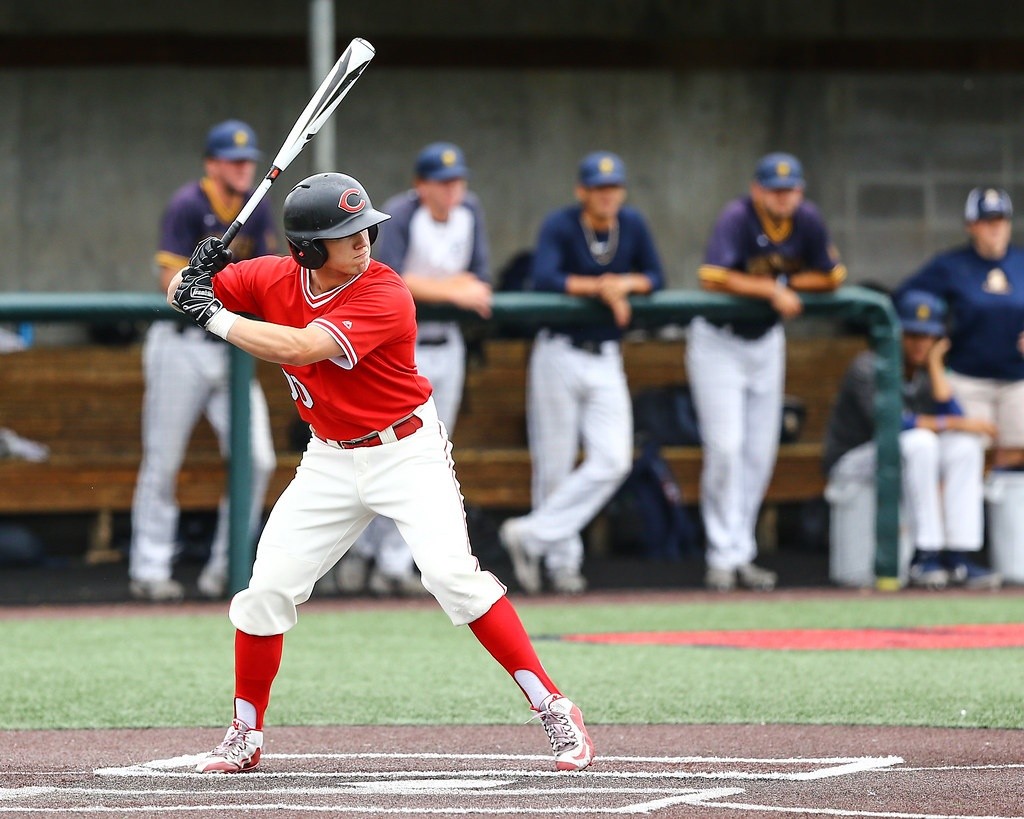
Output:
<box><xmin>897</xmin><ymin>288</ymin><xmax>947</xmax><ymax>337</ymax></box>
<box><xmin>964</xmin><ymin>185</ymin><xmax>1013</xmax><ymax>222</ymax></box>
<box><xmin>205</xmin><ymin>119</ymin><xmax>264</xmax><ymax>163</ymax></box>
<box><xmin>577</xmin><ymin>152</ymin><xmax>627</xmax><ymax>187</ymax></box>
<box><xmin>413</xmin><ymin>141</ymin><xmax>469</xmax><ymax>183</ymax></box>
<box><xmin>755</xmin><ymin>152</ymin><xmax>805</xmax><ymax>191</ymax></box>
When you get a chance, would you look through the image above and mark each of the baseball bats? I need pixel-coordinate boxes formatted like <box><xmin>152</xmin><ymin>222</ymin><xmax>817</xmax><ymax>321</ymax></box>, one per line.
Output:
<box><xmin>171</xmin><ymin>37</ymin><xmax>377</xmax><ymax>315</ymax></box>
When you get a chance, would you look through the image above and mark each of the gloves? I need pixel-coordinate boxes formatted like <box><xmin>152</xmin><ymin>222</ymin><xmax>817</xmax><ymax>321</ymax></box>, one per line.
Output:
<box><xmin>171</xmin><ymin>236</ymin><xmax>233</xmax><ymax>328</ymax></box>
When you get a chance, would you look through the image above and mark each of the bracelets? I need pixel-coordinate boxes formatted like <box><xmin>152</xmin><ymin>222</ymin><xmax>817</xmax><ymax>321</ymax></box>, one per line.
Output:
<box><xmin>623</xmin><ymin>273</ymin><xmax>634</xmax><ymax>292</ymax></box>
<box><xmin>937</xmin><ymin>412</ymin><xmax>945</xmax><ymax>432</ymax></box>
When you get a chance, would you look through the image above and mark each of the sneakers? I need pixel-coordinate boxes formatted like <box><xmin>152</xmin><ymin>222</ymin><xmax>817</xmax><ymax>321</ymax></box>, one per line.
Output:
<box><xmin>524</xmin><ymin>693</ymin><xmax>595</xmax><ymax>772</ymax></box>
<box><xmin>195</xmin><ymin>718</ymin><xmax>264</xmax><ymax>774</ymax></box>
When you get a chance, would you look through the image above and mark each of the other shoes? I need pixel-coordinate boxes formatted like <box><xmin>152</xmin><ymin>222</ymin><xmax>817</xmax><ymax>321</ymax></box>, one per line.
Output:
<box><xmin>546</xmin><ymin>567</ymin><xmax>587</xmax><ymax>594</ymax></box>
<box><xmin>950</xmin><ymin>558</ymin><xmax>1005</xmax><ymax>590</ymax></box>
<box><xmin>704</xmin><ymin>566</ymin><xmax>735</xmax><ymax>591</ymax></box>
<box><xmin>499</xmin><ymin>518</ymin><xmax>541</xmax><ymax>595</ymax></box>
<box><xmin>198</xmin><ymin>573</ymin><xmax>227</xmax><ymax>598</ymax></box>
<box><xmin>910</xmin><ymin>554</ymin><xmax>950</xmax><ymax>594</ymax></box>
<box><xmin>738</xmin><ymin>562</ymin><xmax>778</xmax><ymax>588</ymax></box>
<box><xmin>318</xmin><ymin>559</ymin><xmax>435</xmax><ymax>600</ymax></box>
<box><xmin>128</xmin><ymin>576</ymin><xmax>185</xmax><ymax>601</ymax></box>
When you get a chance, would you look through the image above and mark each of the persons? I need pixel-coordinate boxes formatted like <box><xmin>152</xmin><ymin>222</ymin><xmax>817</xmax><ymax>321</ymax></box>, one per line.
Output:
<box><xmin>127</xmin><ymin>121</ymin><xmax>277</xmax><ymax>600</ymax></box>
<box><xmin>892</xmin><ymin>186</ymin><xmax>1024</xmax><ymax>585</ymax></box>
<box><xmin>684</xmin><ymin>152</ymin><xmax>849</xmax><ymax>589</ymax></box>
<box><xmin>820</xmin><ymin>290</ymin><xmax>1003</xmax><ymax>588</ymax></box>
<box><xmin>167</xmin><ymin>171</ymin><xmax>594</xmax><ymax>774</ymax></box>
<box><xmin>335</xmin><ymin>142</ymin><xmax>493</xmax><ymax>594</ymax></box>
<box><xmin>500</xmin><ymin>151</ymin><xmax>667</xmax><ymax>593</ymax></box>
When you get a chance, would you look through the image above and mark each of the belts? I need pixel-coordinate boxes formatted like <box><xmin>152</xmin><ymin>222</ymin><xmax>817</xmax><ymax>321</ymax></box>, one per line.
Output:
<box><xmin>546</xmin><ymin>331</ymin><xmax>603</xmax><ymax>356</ymax></box>
<box><xmin>176</xmin><ymin>321</ymin><xmax>226</xmax><ymax>344</ymax></box>
<box><xmin>420</xmin><ymin>335</ymin><xmax>448</xmax><ymax>346</ymax></box>
<box><xmin>315</xmin><ymin>414</ymin><xmax>423</xmax><ymax>449</ymax></box>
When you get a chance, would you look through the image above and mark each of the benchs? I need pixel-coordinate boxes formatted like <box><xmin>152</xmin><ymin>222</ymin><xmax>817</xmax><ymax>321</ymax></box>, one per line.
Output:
<box><xmin>1</xmin><ymin>337</ymin><xmax>868</xmax><ymax>562</ymax></box>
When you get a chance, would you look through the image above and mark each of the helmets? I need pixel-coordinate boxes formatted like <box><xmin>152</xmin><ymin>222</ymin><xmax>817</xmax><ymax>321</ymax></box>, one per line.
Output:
<box><xmin>282</xmin><ymin>172</ymin><xmax>393</xmax><ymax>269</ymax></box>
